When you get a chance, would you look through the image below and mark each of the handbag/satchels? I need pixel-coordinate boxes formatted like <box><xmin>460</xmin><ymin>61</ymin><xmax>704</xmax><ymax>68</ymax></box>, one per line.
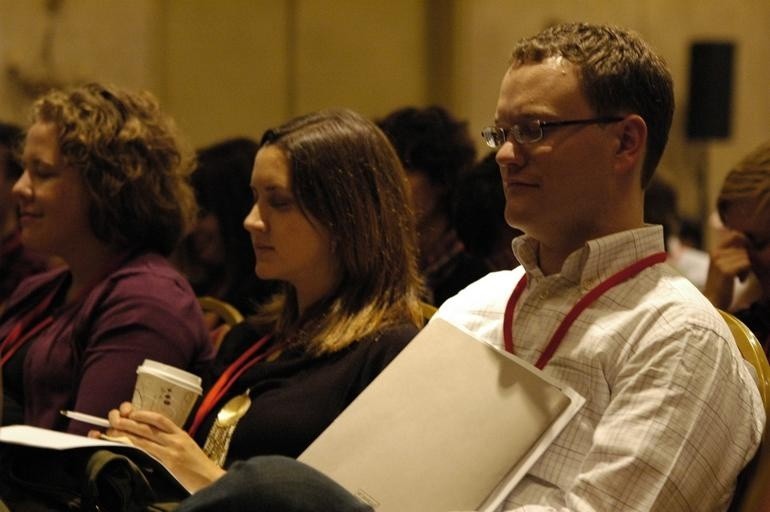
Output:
<box><xmin>0</xmin><ymin>446</ymin><xmax>182</xmax><ymax>512</ymax></box>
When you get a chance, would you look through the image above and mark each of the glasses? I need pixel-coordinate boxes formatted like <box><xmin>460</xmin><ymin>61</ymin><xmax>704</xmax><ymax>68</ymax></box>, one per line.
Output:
<box><xmin>481</xmin><ymin>116</ymin><xmax>624</xmax><ymax>149</ymax></box>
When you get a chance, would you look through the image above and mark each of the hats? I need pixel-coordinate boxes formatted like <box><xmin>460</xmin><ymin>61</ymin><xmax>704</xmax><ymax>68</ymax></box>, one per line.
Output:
<box><xmin>717</xmin><ymin>140</ymin><xmax>770</xmax><ymax>239</ymax></box>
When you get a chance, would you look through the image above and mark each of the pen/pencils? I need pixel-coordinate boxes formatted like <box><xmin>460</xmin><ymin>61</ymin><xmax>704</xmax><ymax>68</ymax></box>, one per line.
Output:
<box><xmin>58</xmin><ymin>409</ymin><xmax>110</xmax><ymax>429</ymax></box>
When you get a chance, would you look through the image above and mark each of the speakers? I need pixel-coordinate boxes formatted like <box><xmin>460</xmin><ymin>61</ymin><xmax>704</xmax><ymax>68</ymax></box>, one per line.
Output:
<box><xmin>686</xmin><ymin>40</ymin><xmax>734</xmax><ymax>140</ymax></box>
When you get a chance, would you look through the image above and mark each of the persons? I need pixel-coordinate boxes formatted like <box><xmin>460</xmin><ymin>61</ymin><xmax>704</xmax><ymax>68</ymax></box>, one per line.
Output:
<box><xmin>1</xmin><ymin>23</ymin><xmax>770</xmax><ymax>511</ymax></box>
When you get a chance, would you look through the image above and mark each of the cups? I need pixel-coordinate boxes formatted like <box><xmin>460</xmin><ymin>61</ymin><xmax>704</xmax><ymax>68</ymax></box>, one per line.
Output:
<box><xmin>131</xmin><ymin>358</ymin><xmax>203</xmax><ymax>429</ymax></box>
